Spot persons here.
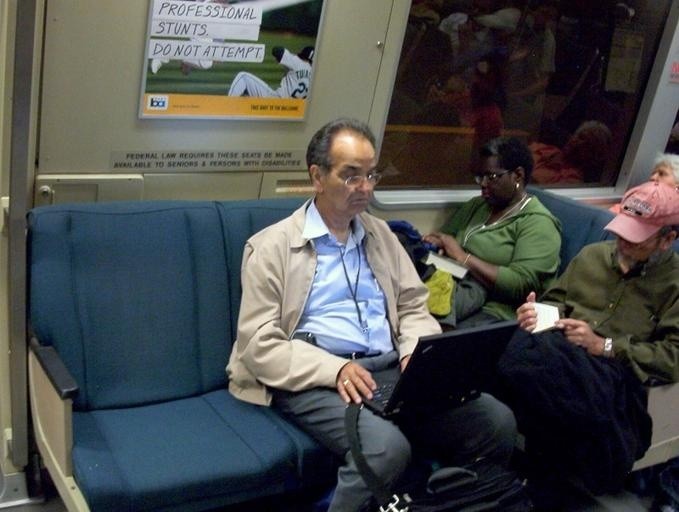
[490,180,679,497]
[227,44,313,100]
[648,152,679,189]
[217,116,525,508]
[412,134,566,334]
[378,0,632,188]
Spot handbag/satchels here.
[367,464,532,512]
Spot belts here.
[335,351,382,360]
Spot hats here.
[476,7,522,31]
[604,181,679,244]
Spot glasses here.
[473,169,513,184]
[327,164,382,189]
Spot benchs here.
[524,187,679,504]
[26,196,335,512]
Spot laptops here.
[362,320,521,420]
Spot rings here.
[342,378,350,386]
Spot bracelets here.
[603,334,613,358]
[462,252,472,266]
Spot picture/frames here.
[136,0,330,123]
